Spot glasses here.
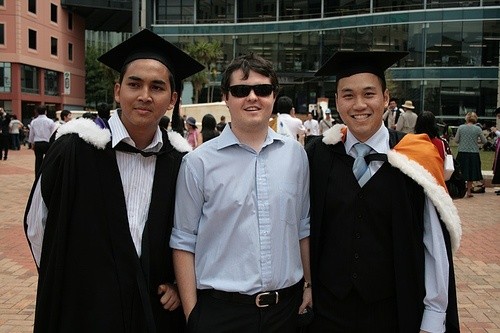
[227,83,277,98]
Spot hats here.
[313,50,411,81]
[97,27,206,84]
[185,117,196,126]
[401,100,415,109]
[325,108,331,113]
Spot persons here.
[0,108,23,160]
[305,50,463,333]
[33,29,206,333]
[269,96,340,148]
[170,50,313,333]
[161,114,227,149]
[491,107,500,195]
[382,98,445,164]
[455,112,487,198]
[28,103,110,178]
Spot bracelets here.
[304,281,312,288]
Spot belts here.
[196,277,306,309]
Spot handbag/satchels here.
[443,153,455,183]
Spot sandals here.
[470,183,486,194]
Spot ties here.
[350,143,374,187]
